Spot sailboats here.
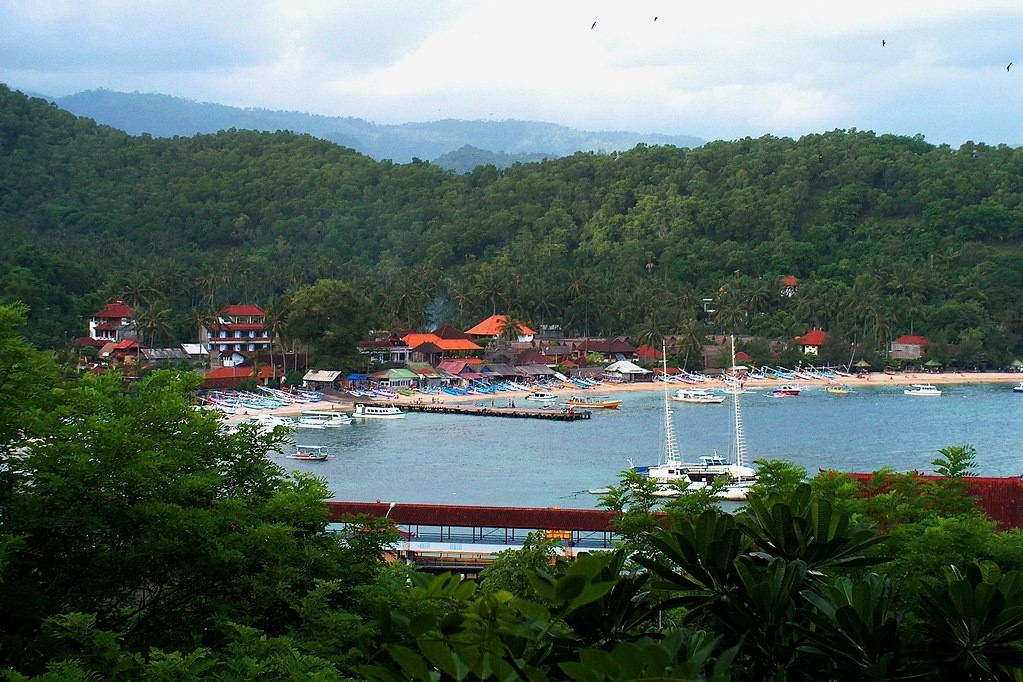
[628,333,772,500]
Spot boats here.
[200,385,354,439]
[762,384,800,399]
[524,391,558,402]
[652,348,857,384]
[1013,380,1023,392]
[587,488,614,494]
[884,369,897,375]
[671,386,727,403]
[351,404,408,419]
[286,444,329,460]
[904,383,941,397]
[825,384,851,394]
[718,385,747,395]
[564,395,622,409]
[559,401,605,412]
[345,374,601,398]
